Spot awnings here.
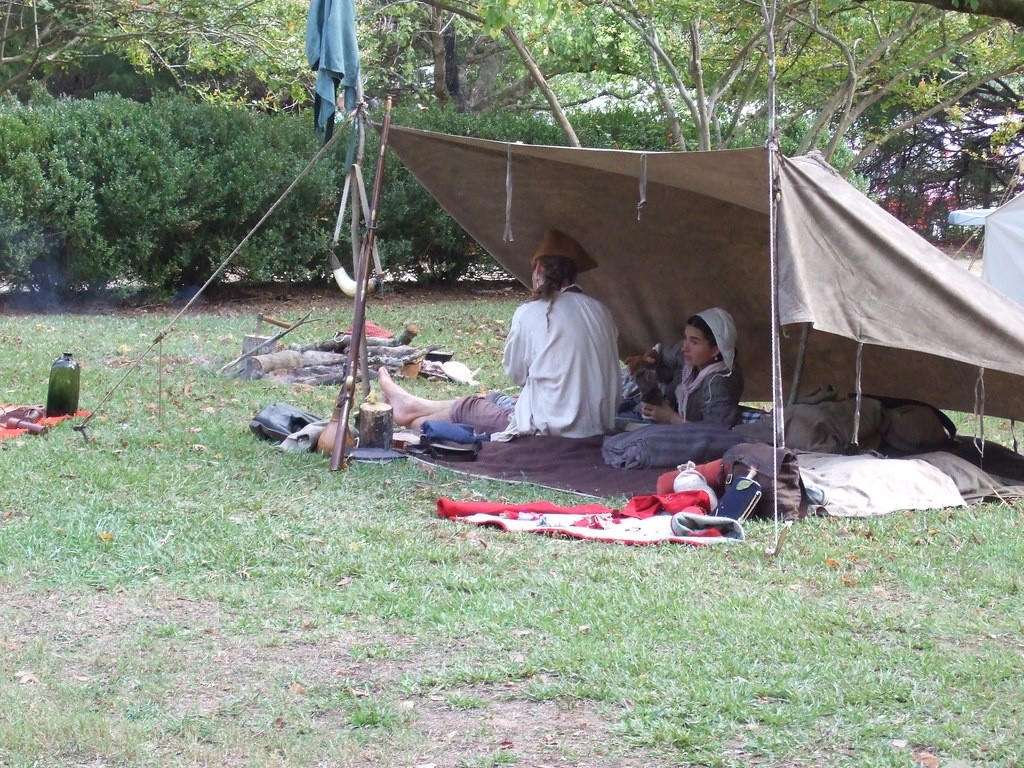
[72,102,1024,557]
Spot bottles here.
[45,352,80,418]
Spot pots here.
[426,351,455,363]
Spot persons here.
[617,307,744,430]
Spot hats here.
[531,229,598,272]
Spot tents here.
[377,230,621,442]
[948,188,1024,308]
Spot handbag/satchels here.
[717,442,808,522]
[249,403,321,443]
[277,418,359,456]
[392,420,482,462]
